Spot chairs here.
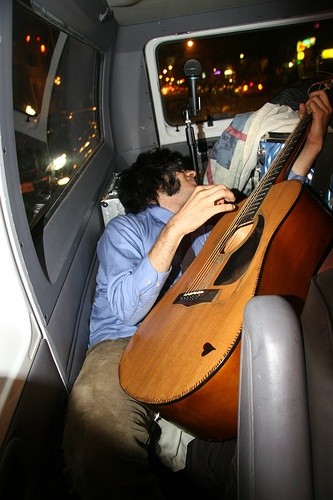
[236,267,333,500]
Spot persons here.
[56,89,333,500]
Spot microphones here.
[185,59,202,116]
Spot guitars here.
[118,81,332,442]
